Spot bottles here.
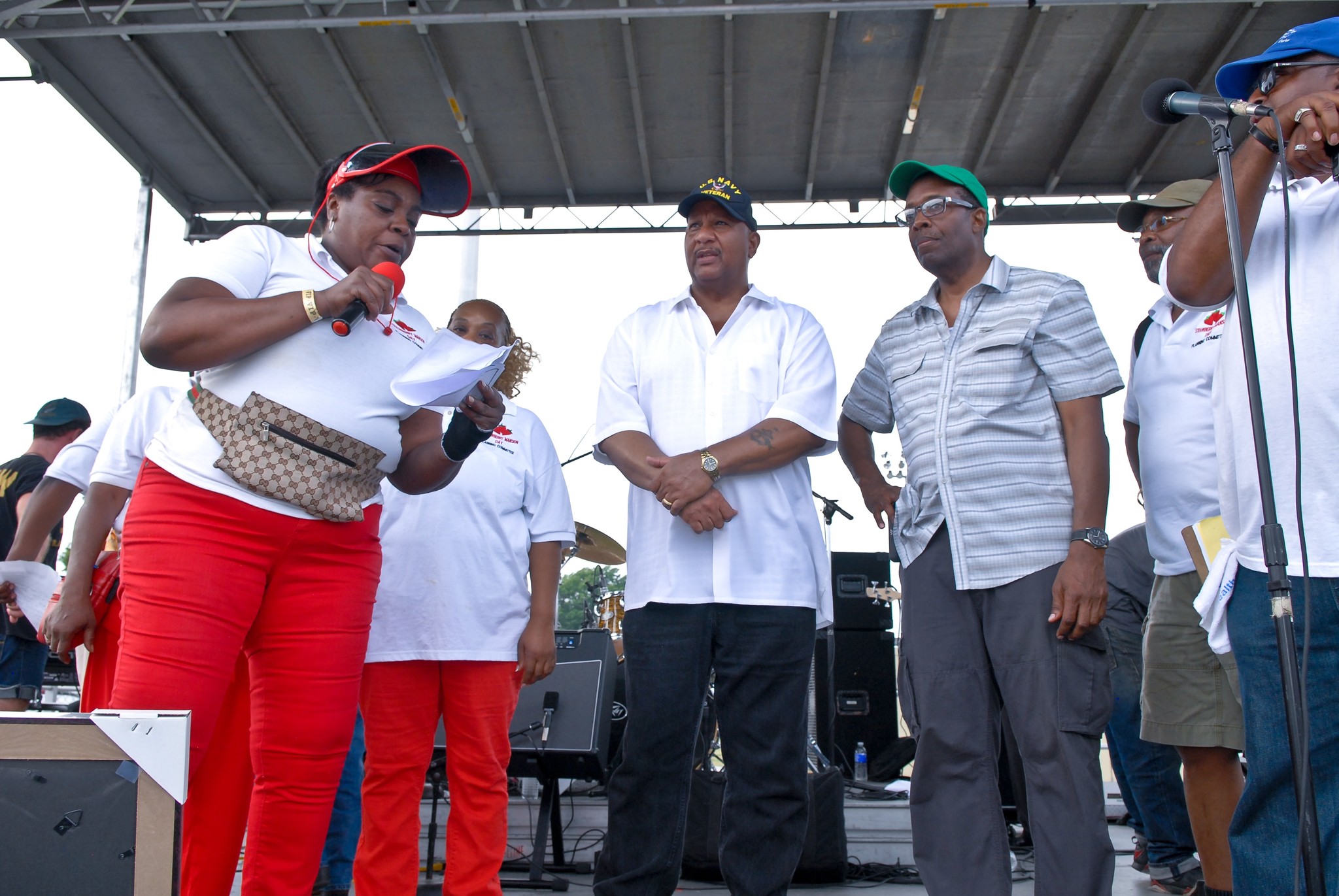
[855,742,868,782]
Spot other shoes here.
[1008,829,1035,848]
[1149,862,1206,893]
[1130,841,1151,874]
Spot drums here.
[607,652,718,771]
[594,589,625,659]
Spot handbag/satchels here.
[187,381,389,523]
[36,547,121,651]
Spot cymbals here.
[562,520,628,566]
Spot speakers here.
[813,630,901,781]
[831,552,894,632]
[688,770,847,883]
[434,629,615,779]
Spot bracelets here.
[1248,123,1287,155]
[302,289,323,323]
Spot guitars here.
[866,580,902,608]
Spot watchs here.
[1070,527,1109,549]
[700,447,720,481]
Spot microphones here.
[331,262,405,337]
[542,691,559,748]
[1140,79,1274,126]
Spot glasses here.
[1244,61,1339,101]
[1132,216,1187,243]
[895,196,973,227]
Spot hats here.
[888,160,989,234]
[23,398,91,426]
[327,141,472,217]
[1215,17,1339,101]
[1116,179,1213,232]
[677,175,757,231]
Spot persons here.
[1101,519,1206,896]
[1159,17,1339,896]
[109,142,505,896]
[1118,178,1248,896]
[837,160,1126,896]
[1,383,180,713]
[352,299,577,896]
[312,704,366,896]
[589,177,839,895]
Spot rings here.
[1294,144,1307,150]
[1294,107,1314,123]
[663,498,672,505]
